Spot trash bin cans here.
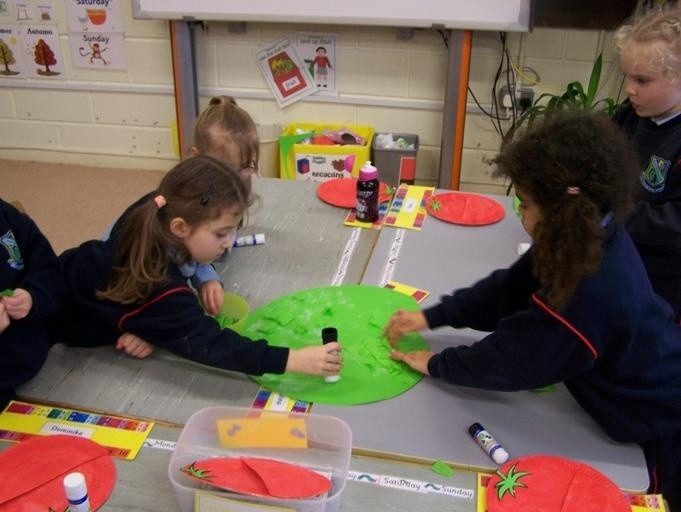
[372,131,419,185]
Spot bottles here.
[355,160,380,222]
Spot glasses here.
[239,160,258,179]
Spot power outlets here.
[499,88,534,110]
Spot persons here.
[0,195,61,417]
[48,155,344,379]
[189,95,259,195]
[599,8,681,316]
[380,105,678,512]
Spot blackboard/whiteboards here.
[131,0,536,33]
